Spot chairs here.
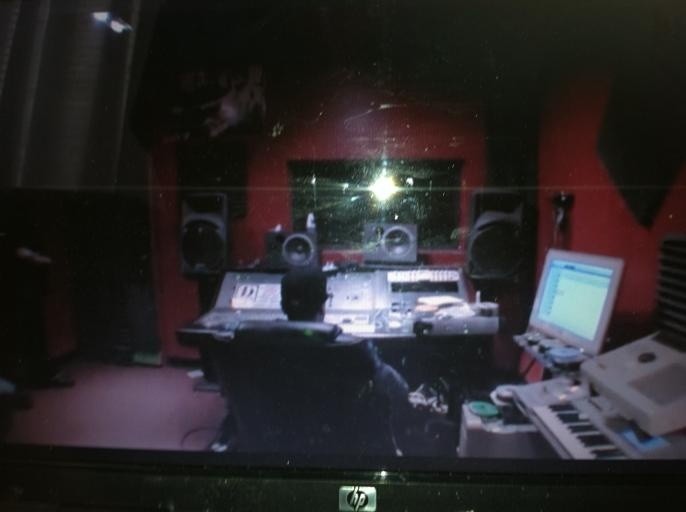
[230,338,368,455]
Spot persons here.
[272,268,408,431]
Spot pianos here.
[530,403,640,459]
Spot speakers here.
[267,228,322,272]
[363,223,419,265]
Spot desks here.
[514,330,577,460]
[178,326,495,400]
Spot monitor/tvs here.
[529,248,626,357]
[0,1,686,511]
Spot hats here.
[281,267,327,313]
